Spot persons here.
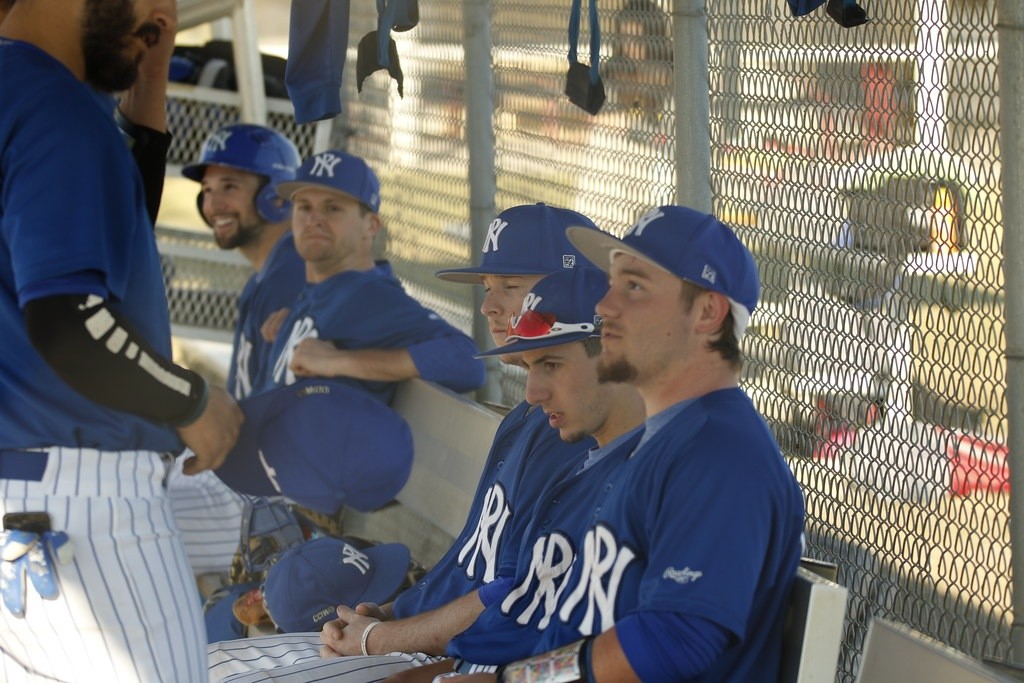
[433,208,803,682]
[389,272,648,683]
[0,0,246,683]
[167,149,484,576]
[181,123,302,402]
[207,201,601,679]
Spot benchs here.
[197,376,850,683]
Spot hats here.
[263,536,410,633]
[435,201,600,284]
[471,265,611,360]
[273,149,381,213]
[564,205,761,342]
[213,377,414,516]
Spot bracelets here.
[361,621,384,657]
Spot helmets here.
[181,124,302,229]
